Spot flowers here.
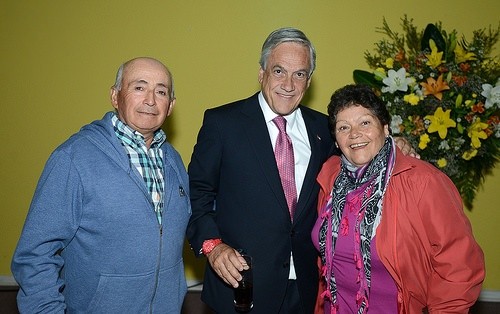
[351,14,500,208]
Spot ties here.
[272,115,298,223]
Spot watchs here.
[202,239,222,254]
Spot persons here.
[311,82,487,314]
[12,57,216,314]
[188,27,420,314]
